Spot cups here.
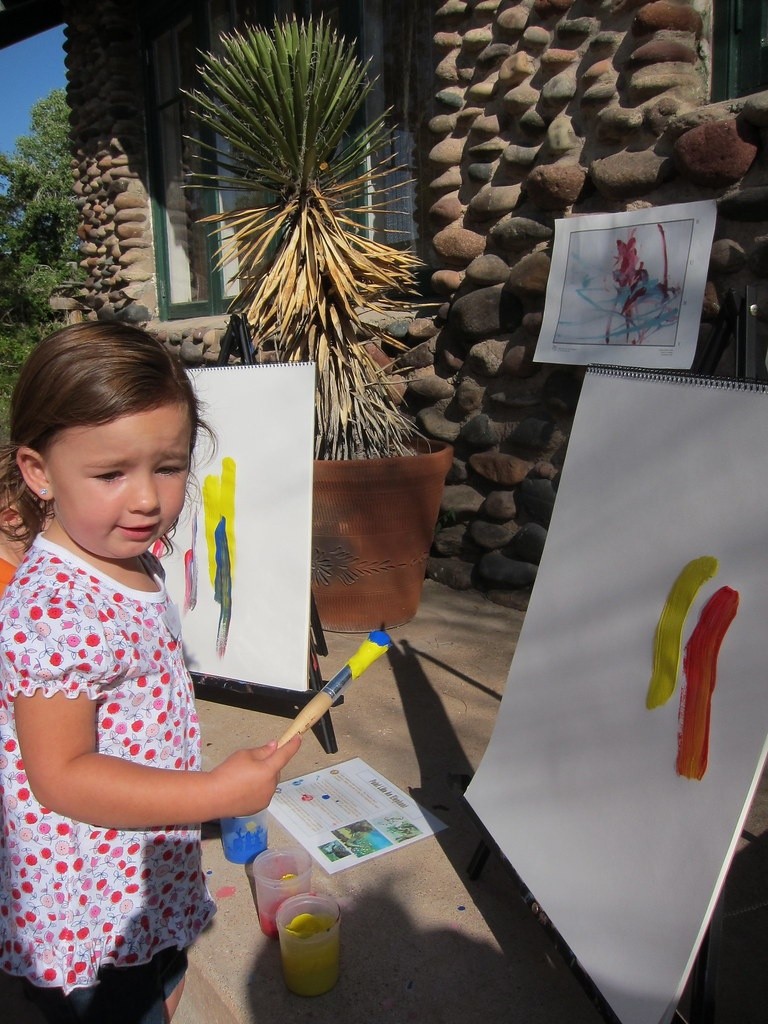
[275,892,343,997]
[219,807,270,864]
[252,846,313,940]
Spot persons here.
[0,319,304,1024]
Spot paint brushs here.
[276,632,392,748]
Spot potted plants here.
[175,10,452,631]
